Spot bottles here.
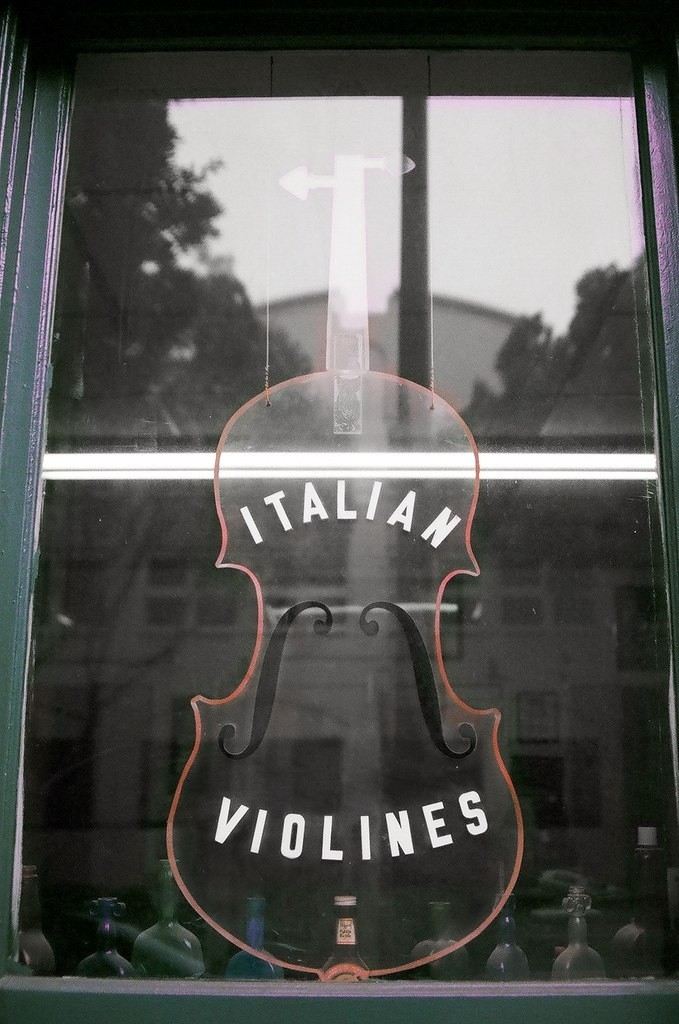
[132,856,206,981]
[316,895,376,983]
[406,901,475,983]
[222,896,285,980]
[605,828,679,981]
[483,892,533,984]
[75,896,137,980]
[550,884,609,980]
[17,864,57,977]
